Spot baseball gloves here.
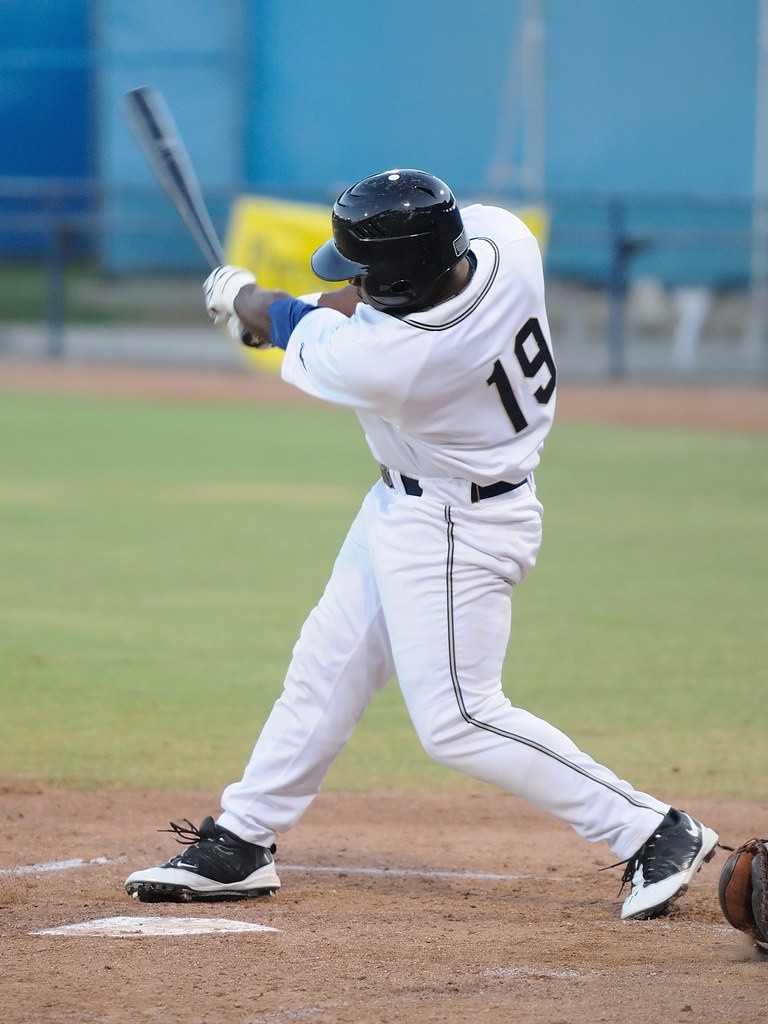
[717,836,768,950]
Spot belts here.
[379,463,527,502]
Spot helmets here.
[311,169,470,306]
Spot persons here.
[126,171,720,920]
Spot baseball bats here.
[125,84,268,349]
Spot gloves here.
[203,264,255,324]
[222,313,276,349]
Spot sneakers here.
[125,816,281,902]
[599,808,720,920]
